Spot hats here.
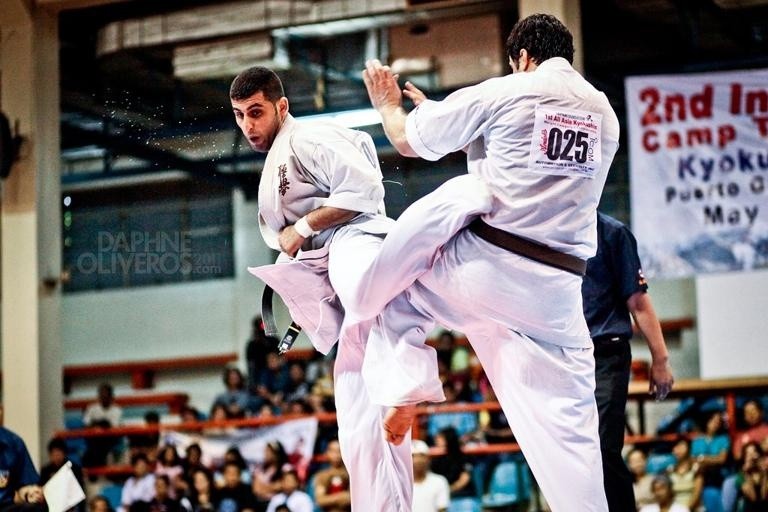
[408,439,432,458]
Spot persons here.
[362,13,621,512]
[36,315,352,512]
[625,399,767,512]
[0,397,42,511]
[230,67,494,512]
[582,208,673,511]
[406,352,530,512]
